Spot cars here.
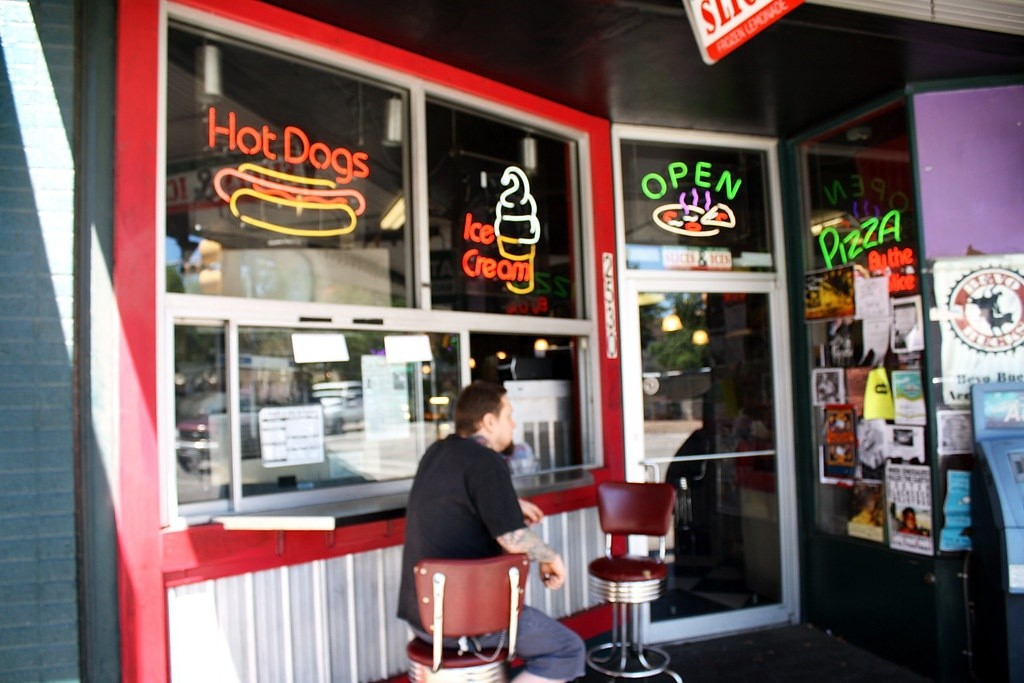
[311,381,365,437]
[179,395,288,465]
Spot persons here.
[665,394,719,561]
[395,382,586,683]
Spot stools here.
[405,554,530,683]
[585,482,683,683]
